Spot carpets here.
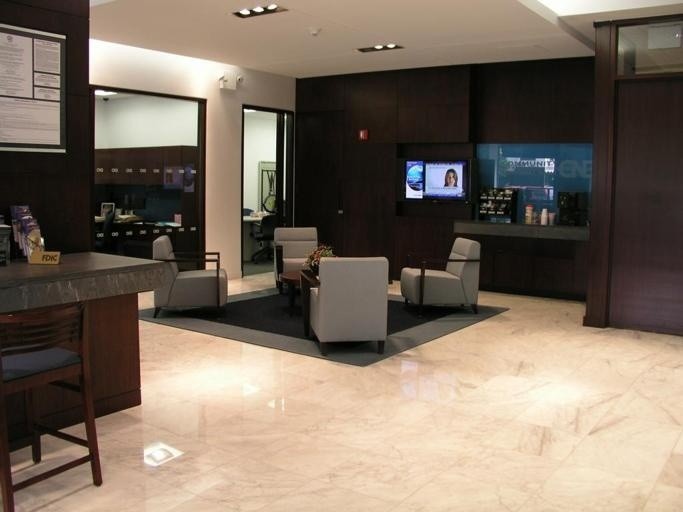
[138,282,511,368]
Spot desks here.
[452,218,589,299]
[243,216,263,262]
[1,247,174,436]
[278,269,309,317]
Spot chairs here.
[248,215,282,265]
[0,303,104,512]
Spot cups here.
[547,212,555,224]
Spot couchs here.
[297,255,393,355]
[149,233,228,321]
[271,226,318,278]
[398,237,486,317]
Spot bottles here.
[525,205,540,224]
[541,207,547,225]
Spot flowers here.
[303,245,335,266]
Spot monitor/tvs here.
[397,159,471,203]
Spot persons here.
[444,168,458,187]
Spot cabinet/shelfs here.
[94,222,197,259]
[94,144,195,189]
